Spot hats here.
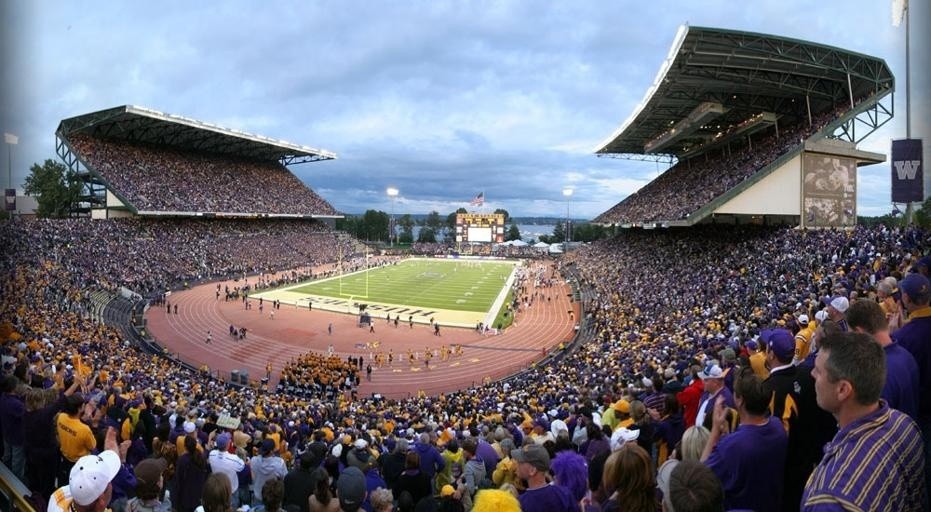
[69,449,122,506]
[133,457,169,485]
[610,426,641,454]
[337,465,367,509]
[759,328,797,357]
[795,301,829,325]
[642,360,690,388]
[509,444,550,471]
[216,432,232,450]
[819,293,851,314]
[718,340,758,364]
[897,272,930,296]
[695,364,724,381]
[5,337,630,441]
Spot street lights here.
[387,185,400,250]
[3,130,19,189]
[890,2,918,229]
[563,184,575,243]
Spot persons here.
[1,91,931,512]
